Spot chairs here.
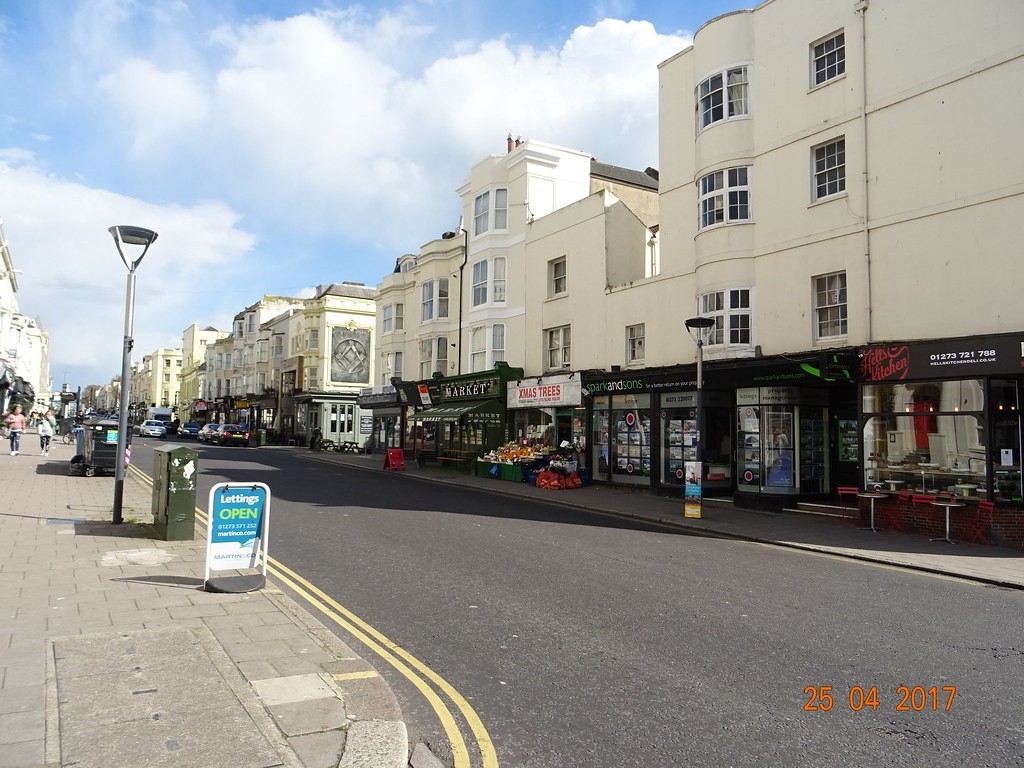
[879,489,939,539]
[957,502,996,546]
[838,486,864,526]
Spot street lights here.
[107,224,159,523]
[684,316,717,519]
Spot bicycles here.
[62,427,75,446]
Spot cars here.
[211,423,251,448]
[176,422,201,439]
[196,422,220,442]
[138,419,168,437]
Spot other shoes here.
[16,450,19,455]
[9,451,14,456]
[45,453,48,457]
[41,449,44,456]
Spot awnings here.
[408,399,505,470]
[183,399,207,410]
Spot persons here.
[74,410,84,439]
[29,411,37,428]
[38,411,56,457]
[309,426,323,449]
[39,411,44,420]
[4,404,25,456]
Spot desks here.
[857,493,890,533]
[321,439,336,451]
[927,501,967,545]
[439,450,478,473]
[343,441,361,454]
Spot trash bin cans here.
[81,417,133,477]
[256,429,266,445]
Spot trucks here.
[147,407,177,434]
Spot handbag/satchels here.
[38,423,43,434]
[4,426,11,437]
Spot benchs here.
[437,455,475,473]
[319,444,363,451]
